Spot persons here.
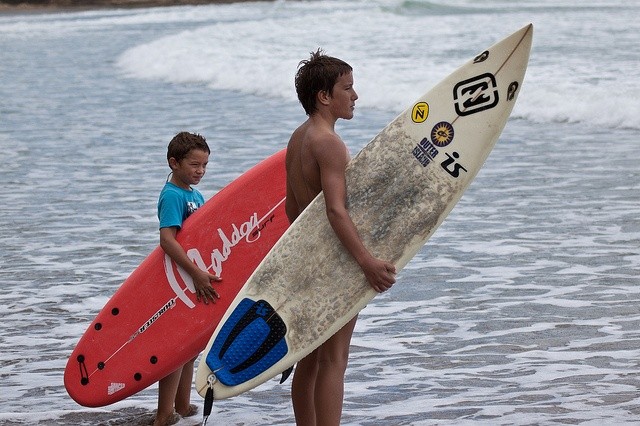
[284,47,397,426]
[153,131,222,425]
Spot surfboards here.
[63,148,290,408]
[195,22,534,399]
